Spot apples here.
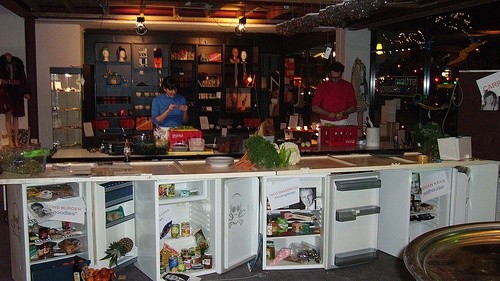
[87,267,116,281]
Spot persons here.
[312,62,357,125]
[0,52,30,149]
[299,188,316,211]
[230,48,247,63]
[30,202,55,223]
[116,47,126,61]
[482,90,498,111]
[151,76,190,143]
[411,173,422,195]
[102,47,110,61]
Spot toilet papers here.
[394,99,400,109]
[381,106,395,122]
[160,127,172,140]
[366,128,379,147]
[385,100,396,106]
[380,124,386,136]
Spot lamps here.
[234,17,248,37]
[134,15,149,37]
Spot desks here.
[50,146,245,162]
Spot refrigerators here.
[259,172,382,270]
[8,182,138,281]
[378,162,500,258]
[134,177,258,281]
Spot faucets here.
[122,128,131,162]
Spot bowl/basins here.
[119,118,135,130]
[206,157,233,168]
[92,120,110,133]
[403,221,500,281]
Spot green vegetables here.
[178,126,193,130]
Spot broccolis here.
[273,142,301,165]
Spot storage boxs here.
[436,135,473,161]
[120,119,134,129]
[243,118,259,128]
[318,125,359,147]
[153,57,163,69]
[135,116,153,130]
[167,129,203,150]
[187,52,195,60]
[188,137,205,151]
[179,49,188,60]
[92,120,110,131]
[208,52,222,62]
[138,57,148,67]
[379,98,406,144]
[138,47,147,57]
[217,118,234,126]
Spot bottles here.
[171,45,193,87]
[119,48,125,62]
[135,114,153,130]
[102,47,109,61]
[106,72,122,86]
[196,71,223,100]
[134,91,161,111]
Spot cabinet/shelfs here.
[49,66,85,149]
[0,150,500,281]
[93,41,308,134]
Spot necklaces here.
[8,60,12,64]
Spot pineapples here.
[100,238,133,268]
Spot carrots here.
[232,151,256,171]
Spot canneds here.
[35,243,44,260]
[272,221,278,235]
[39,226,48,240]
[171,224,180,238]
[204,253,212,269]
[159,183,175,197]
[182,256,191,270]
[181,221,190,237]
